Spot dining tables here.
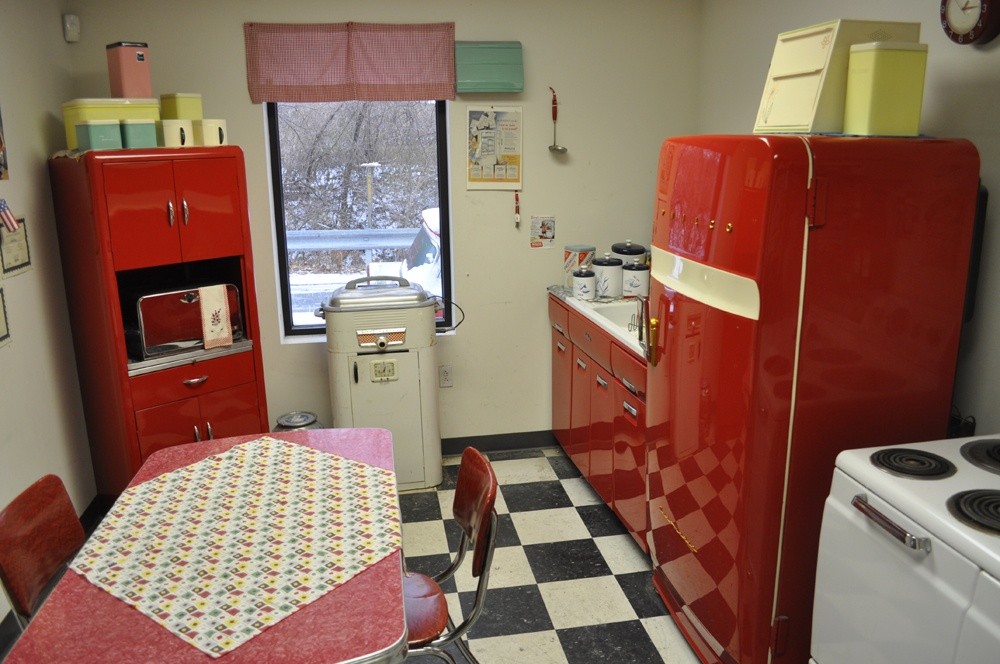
[2,429,410,662]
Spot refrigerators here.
[640,101,978,663]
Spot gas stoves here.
[835,433,999,582]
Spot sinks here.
[566,295,649,360]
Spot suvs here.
[398,207,445,322]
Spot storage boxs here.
[752,18,929,137]
[60,40,227,152]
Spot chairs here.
[404,445,499,664]
[1,473,86,636]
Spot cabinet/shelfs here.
[546,286,647,555]
[45,148,271,511]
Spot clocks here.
[937,1,1000,44]
